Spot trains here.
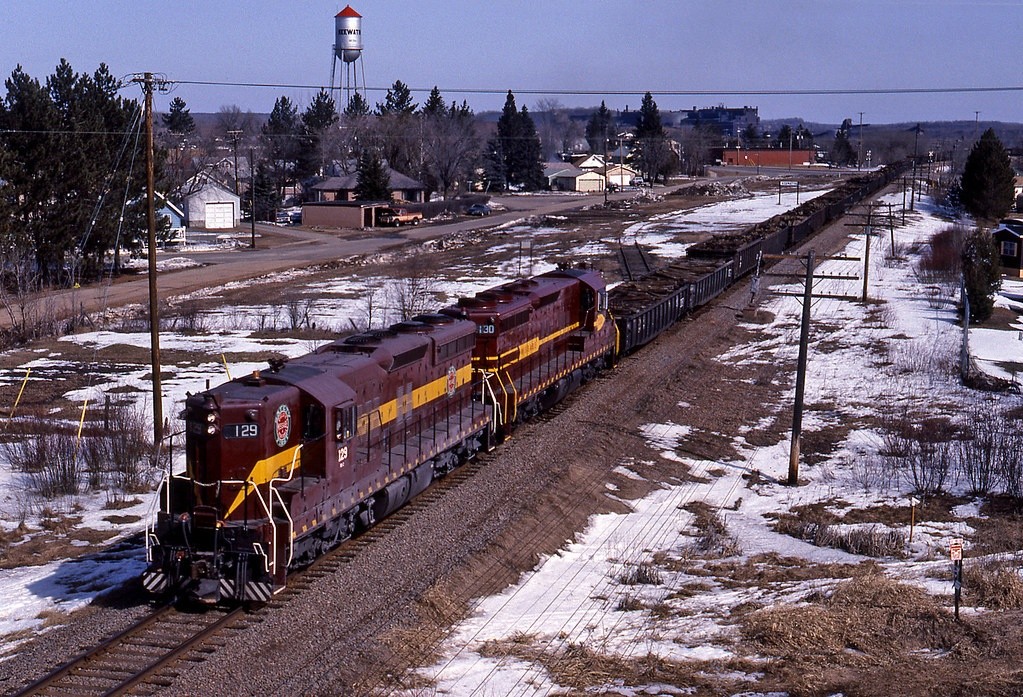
[132,155,947,611]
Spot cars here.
[290,212,302,223]
[275,212,290,223]
[467,204,492,216]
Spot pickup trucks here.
[381,208,423,227]
[629,177,649,186]
